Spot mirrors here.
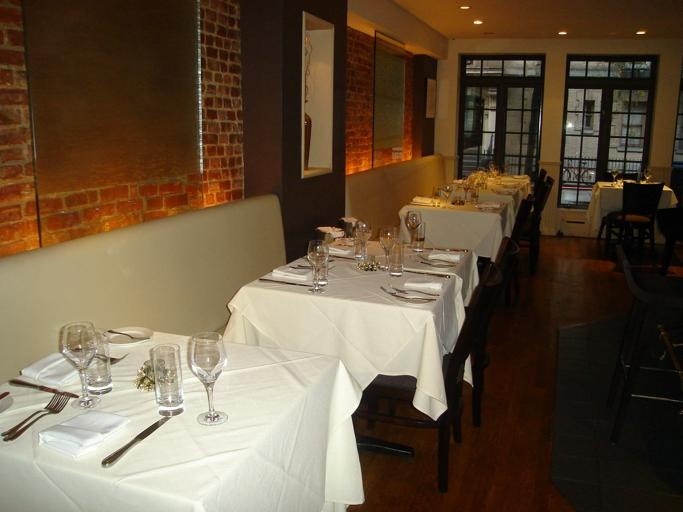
[18,1,205,195]
[370,27,410,154]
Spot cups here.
[85,336,114,394]
[150,340,184,417]
[431,163,502,207]
[413,224,425,253]
[389,237,405,276]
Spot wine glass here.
[187,330,225,425]
[609,167,655,188]
[58,320,100,409]
[295,209,426,294]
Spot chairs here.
[588,168,683,465]
[491,153,557,313]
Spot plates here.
[104,327,155,347]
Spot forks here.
[107,330,149,340]
[2,390,71,445]
[92,349,129,366]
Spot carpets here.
[551,311,682,507]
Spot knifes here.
[95,412,174,467]
[8,379,79,399]
[258,277,314,290]
